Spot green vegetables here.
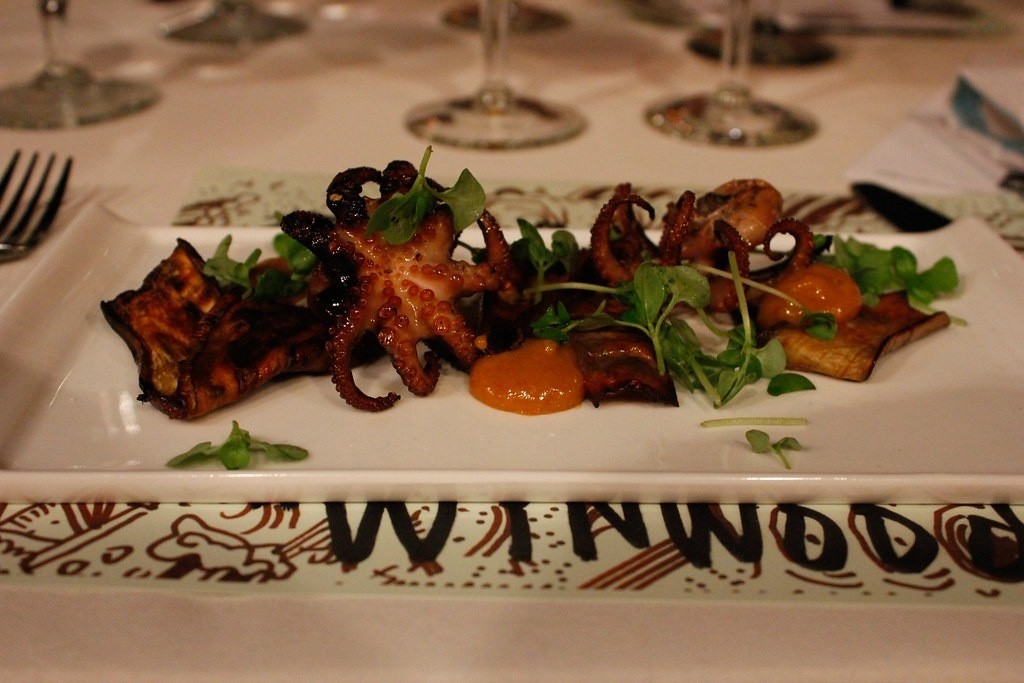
[165,145,973,474]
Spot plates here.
[0,203,1024,505]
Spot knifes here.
[851,181,953,233]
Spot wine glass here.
[646,1,820,149]
[405,0,588,150]
[442,0,569,33]
[689,0,837,68]
[167,0,307,46]
[1,0,157,129]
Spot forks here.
[0,147,74,265]
